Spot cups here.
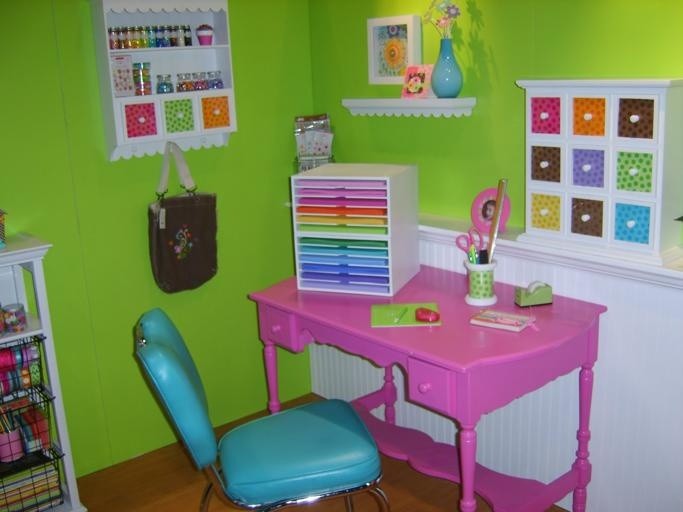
[0,432,22,463]
[196,30,212,45]
[462,258,497,301]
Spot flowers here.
[420,2,464,41]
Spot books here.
[0,455,64,512]
[294,179,389,292]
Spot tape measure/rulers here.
[487,180,506,263]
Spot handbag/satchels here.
[148,193,218,293]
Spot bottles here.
[176,69,223,93]
[132,61,152,96]
[107,23,192,49]
[1,304,28,333]
[156,73,173,93]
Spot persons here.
[483,201,495,222]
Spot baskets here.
[0,334,65,512]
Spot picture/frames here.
[363,10,421,88]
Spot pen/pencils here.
[395,308,407,322]
[469,245,476,264]
[0,406,14,434]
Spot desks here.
[241,264,609,511]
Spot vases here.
[427,37,465,98]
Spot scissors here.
[456,227,483,255]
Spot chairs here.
[128,307,395,512]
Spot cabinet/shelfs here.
[2,230,91,512]
[287,162,423,298]
[510,75,680,268]
[91,2,237,164]
